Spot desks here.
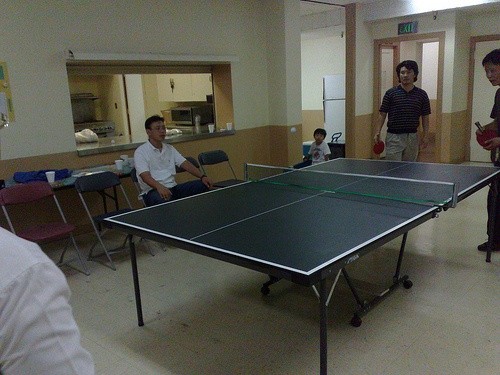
[5,165,120,229]
[102,156,500,375]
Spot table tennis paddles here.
[373,134,385,154]
[475,121,496,147]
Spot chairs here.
[0,180,90,277]
[74,171,154,271]
[176,157,201,173]
[198,150,247,187]
[132,167,166,252]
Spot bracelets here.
[201,175,207,181]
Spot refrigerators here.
[322,74,344,141]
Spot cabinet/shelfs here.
[156,73,212,102]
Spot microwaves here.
[169,105,212,126]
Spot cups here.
[120,155,128,165]
[208,125,214,133]
[45,172,55,183]
[226,123,232,130]
[114,160,123,170]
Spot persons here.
[302,128,332,166]
[133,115,215,207]
[0,226,95,375]
[372,59,432,162]
[475,48,500,252]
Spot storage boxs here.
[302,140,314,156]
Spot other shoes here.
[478,241,500,251]
